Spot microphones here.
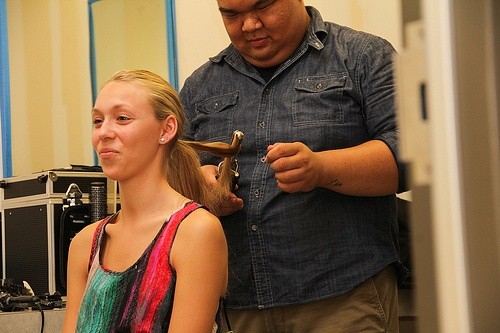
[23,279,42,312]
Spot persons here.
[176,0,409,333]
[62,69,230,333]
